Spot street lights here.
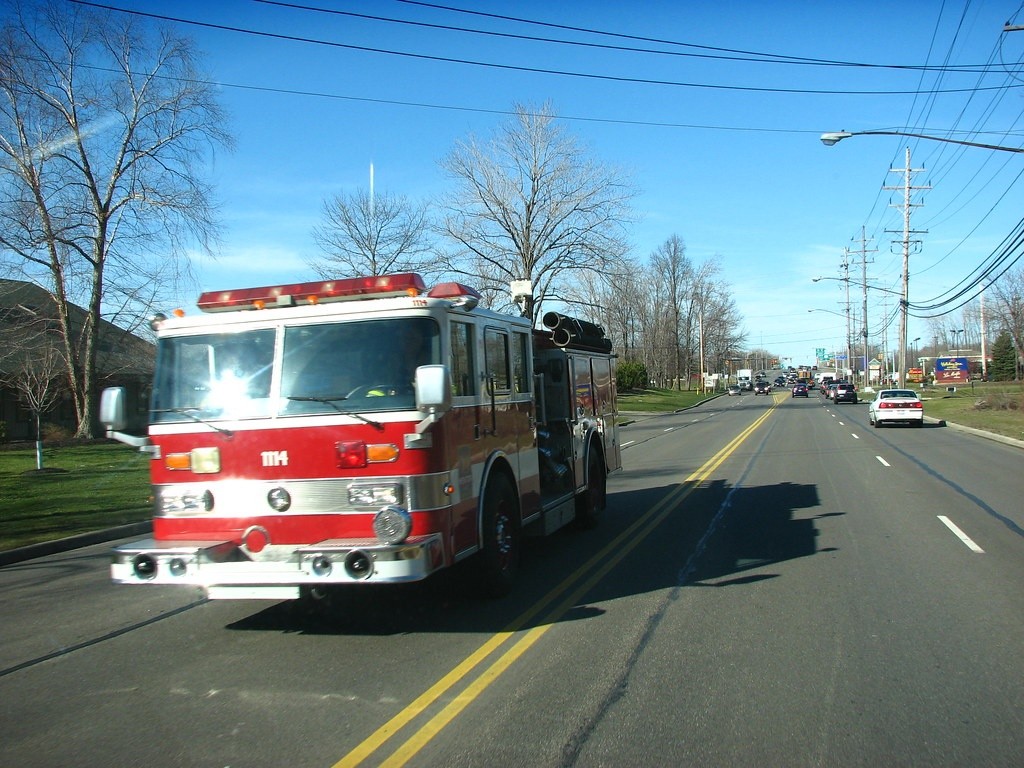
[950,329,964,356]
[812,277,905,390]
[808,307,870,392]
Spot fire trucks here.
[97,272,626,627]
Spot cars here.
[833,384,857,405]
[727,364,848,399]
[868,389,923,428]
